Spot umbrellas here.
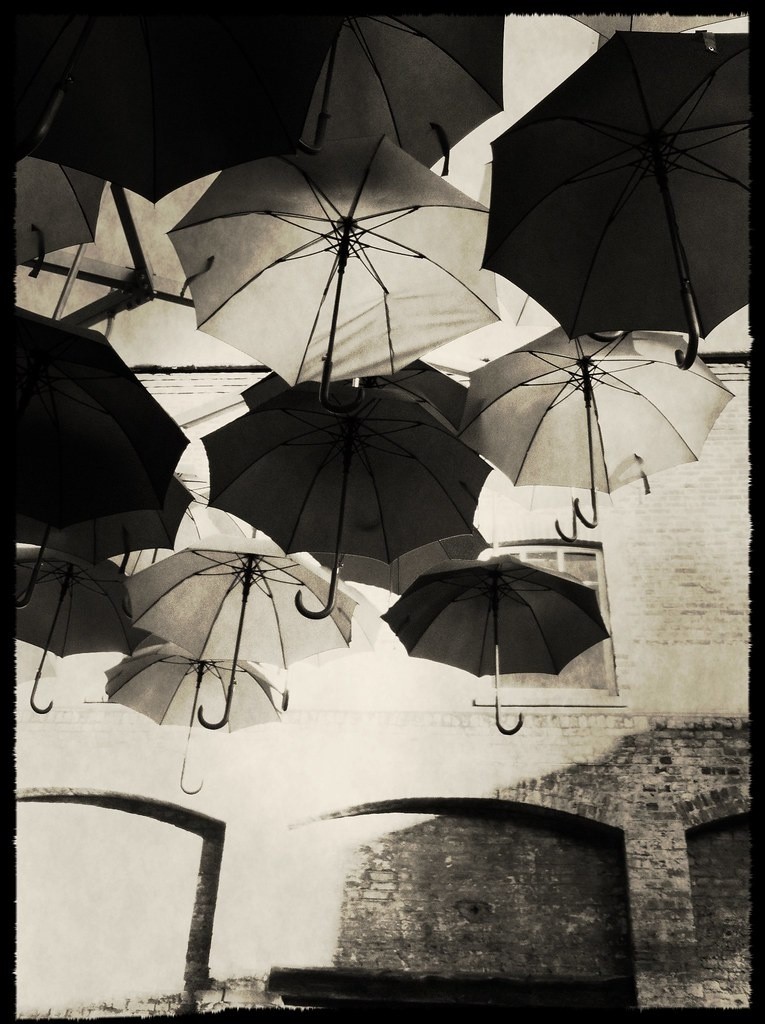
[13,13,506,342]
[202,359,494,620]
[12,302,361,795]
[457,326,734,542]
[381,557,609,734]
[483,15,750,368]
[167,132,500,414]
